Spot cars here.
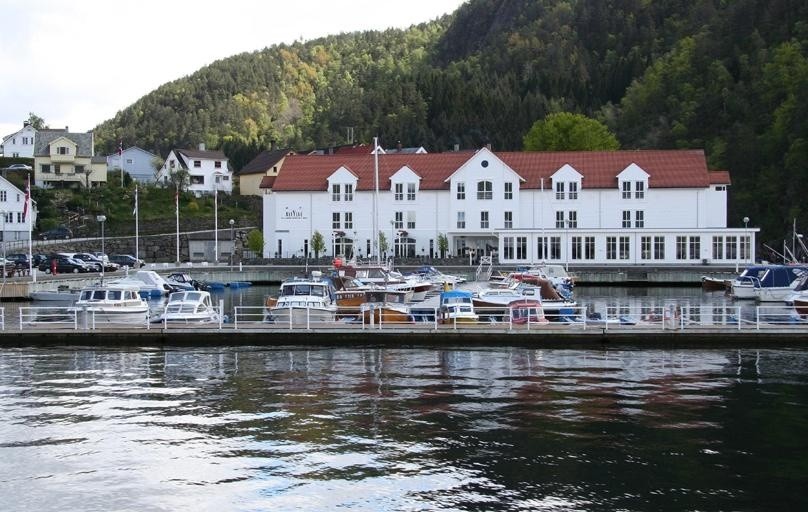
[0,251,146,277]
[38,228,73,241]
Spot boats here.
[28,270,252,323]
[700,217,807,320]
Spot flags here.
[24,174,31,222]
[131,184,137,216]
[114,136,122,158]
[174,185,178,216]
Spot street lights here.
[100,214,107,274]
[391,219,395,272]
[742,217,749,270]
[566,219,569,273]
[229,218,235,266]
